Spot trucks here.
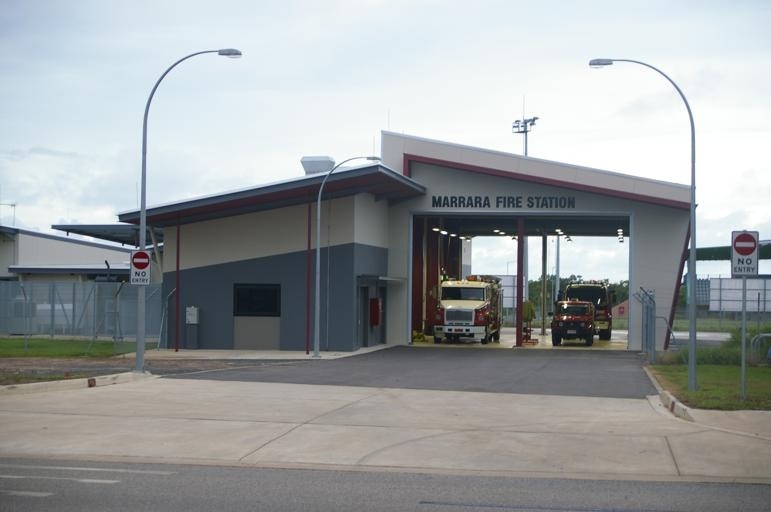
[563,280,616,339]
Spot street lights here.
[132,47,246,374]
[511,116,539,156]
[310,154,382,361]
[587,56,701,397]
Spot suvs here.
[548,301,596,348]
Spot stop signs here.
[732,233,757,258]
[130,251,151,270]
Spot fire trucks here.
[431,275,505,344]
